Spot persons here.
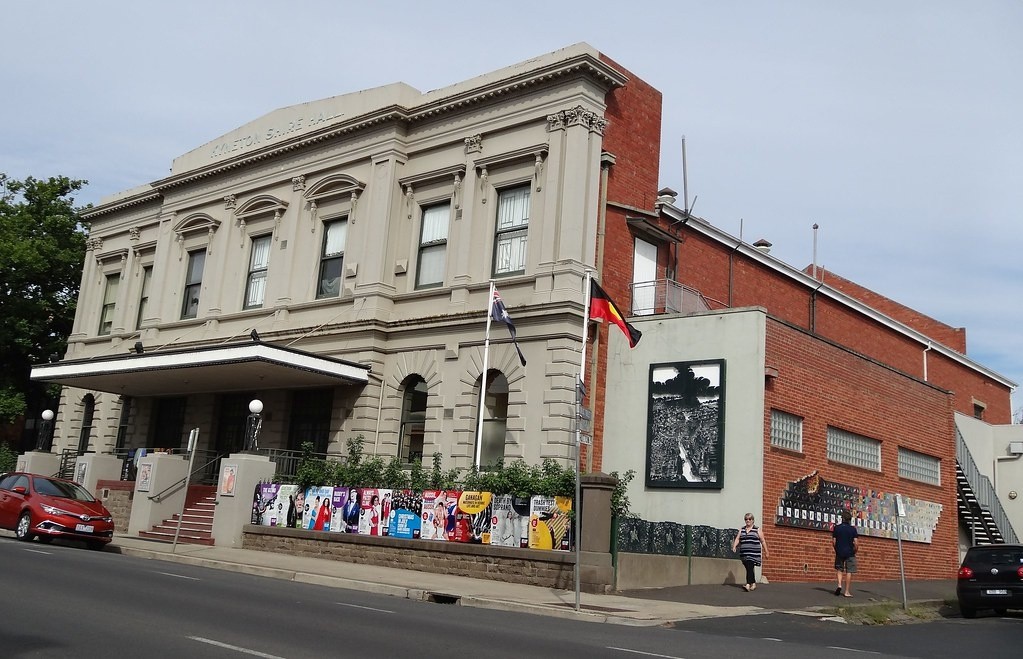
[732,512,769,592]
[832,512,858,596]
[256,489,514,546]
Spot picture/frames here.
[645,358,727,490]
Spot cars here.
[0,472,115,550]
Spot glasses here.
[745,518,752,521]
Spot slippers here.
[845,591,854,597]
[836,587,842,596]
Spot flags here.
[588,277,642,349]
[492,289,527,366]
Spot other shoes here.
[742,585,750,592]
[751,586,757,591]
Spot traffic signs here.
[580,379,587,397]
[579,419,593,446]
[579,405,592,420]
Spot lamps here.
[243,399,263,455]
[250,329,260,341]
[33,410,54,453]
[135,342,144,353]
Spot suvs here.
[956,543,1023,619]
